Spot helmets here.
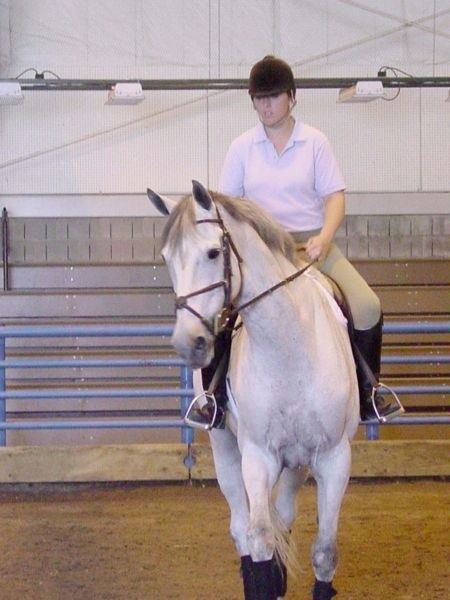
[248,55,294,97]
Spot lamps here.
[0,82,25,105]
[335,81,383,105]
[105,82,146,106]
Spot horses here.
[146,179,360,600]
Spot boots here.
[350,310,401,422]
[188,304,239,429]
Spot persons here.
[188,56,401,429]
